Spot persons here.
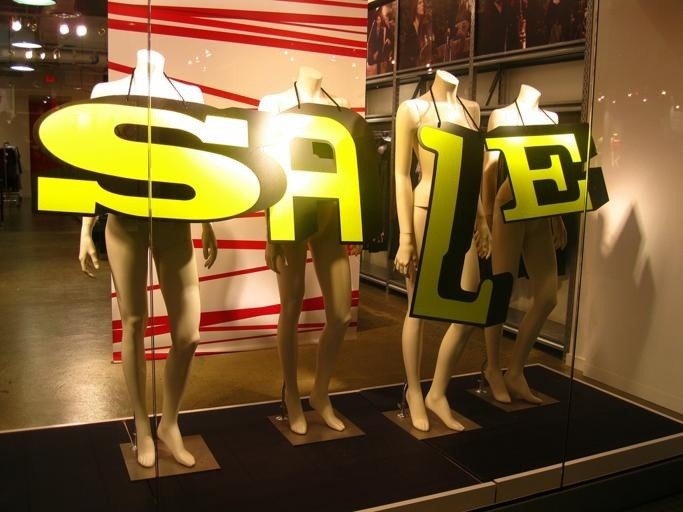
[472,82,570,406]
[252,65,356,437]
[388,65,494,434]
[367,1,576,76]
[69,50,200,471]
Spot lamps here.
[0,0,87,72]
